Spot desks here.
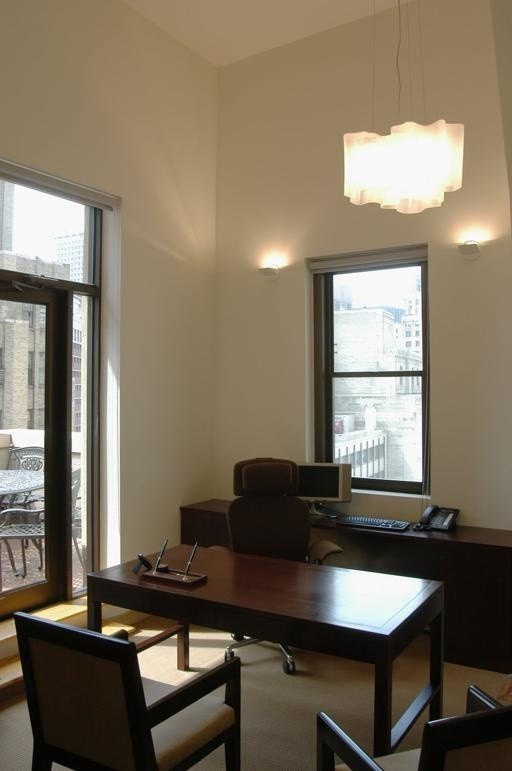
[87,544,442,758]
[179,498,512,674]
[0,469,45,551]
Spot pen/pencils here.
[184,538,199,578]
[153,537,169,575]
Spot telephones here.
[412,503,459,532]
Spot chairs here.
[316,684,512,771]
[0,469,83,577]
[2,445,44,548]
[220,495,343,674]
[15,611,242,771]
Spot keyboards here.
[335,516,411,532]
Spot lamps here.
[344,0,465,214]
[259,265,280,279]
[458,240,481,258]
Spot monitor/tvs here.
[295,463,352,518]
